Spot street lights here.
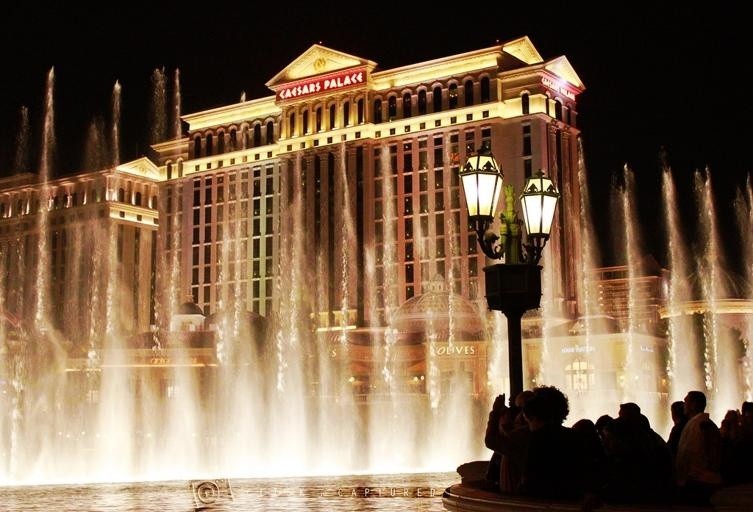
[459,146,562,412]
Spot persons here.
[482,383,752,512]
